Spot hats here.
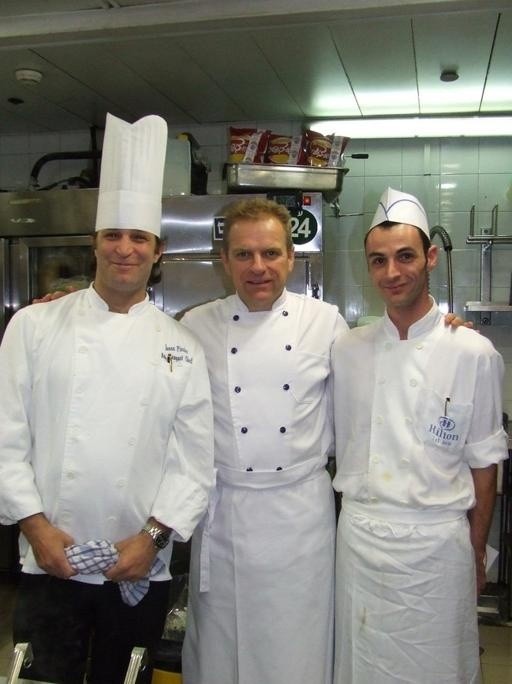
[368,186,431,242]
[96,111,170,241]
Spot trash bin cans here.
[152,574,189,684]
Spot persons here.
[31,196,482,684]
[328,220,510,684]
[0,227,217,684]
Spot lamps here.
[307,110,512,140]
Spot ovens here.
[1,189,97,325]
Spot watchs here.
[143,522,170,548]
